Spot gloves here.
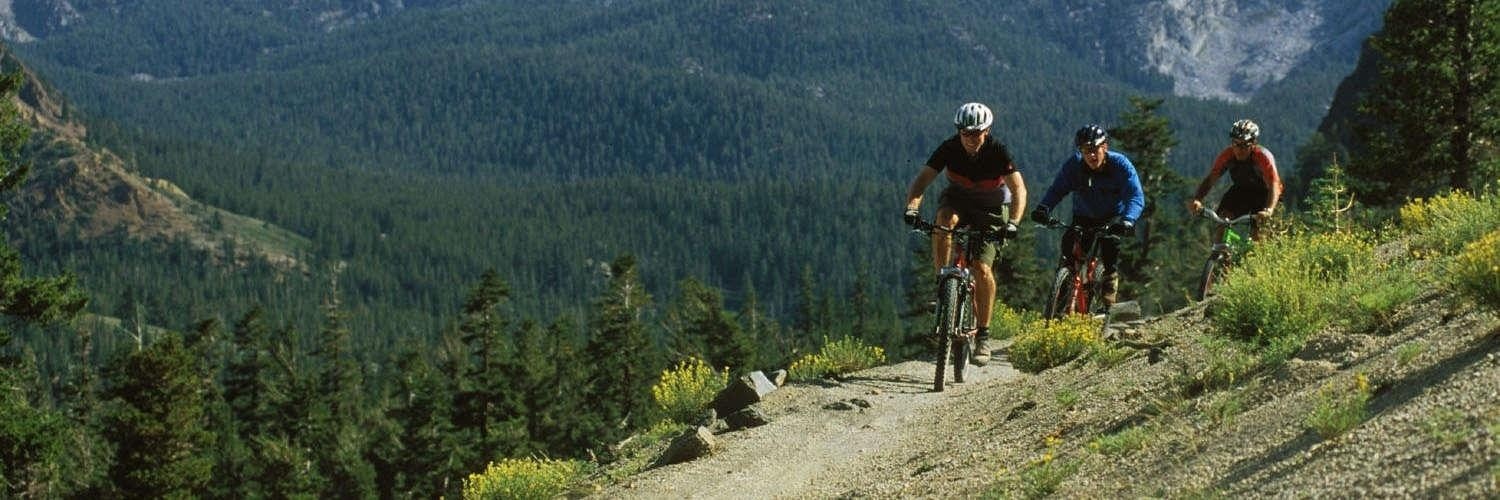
[1003,223,1018,238]
[1032,207,1047,223]
[1257,208,1272,226]
[1107,224,1136,237]
[904,208,918,224]
[1189,198,1201,217]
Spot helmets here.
[1074,124,1107,146]
[954,102,993,132]
[1230,119,1259,140]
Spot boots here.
[1102,273,1118,304]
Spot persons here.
[1031,121,1146,318]
[905,99,1029,368]
[1185,118,1284,267]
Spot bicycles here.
[915,214,1006,392]
[1197,205,1259,301]
[1042,214,1123,340]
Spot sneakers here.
[926,287,939,311]
[970,337,991,367]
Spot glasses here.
[1078,147,1098,153]
[1232,140,1252,147]
[960,133,982,136]
